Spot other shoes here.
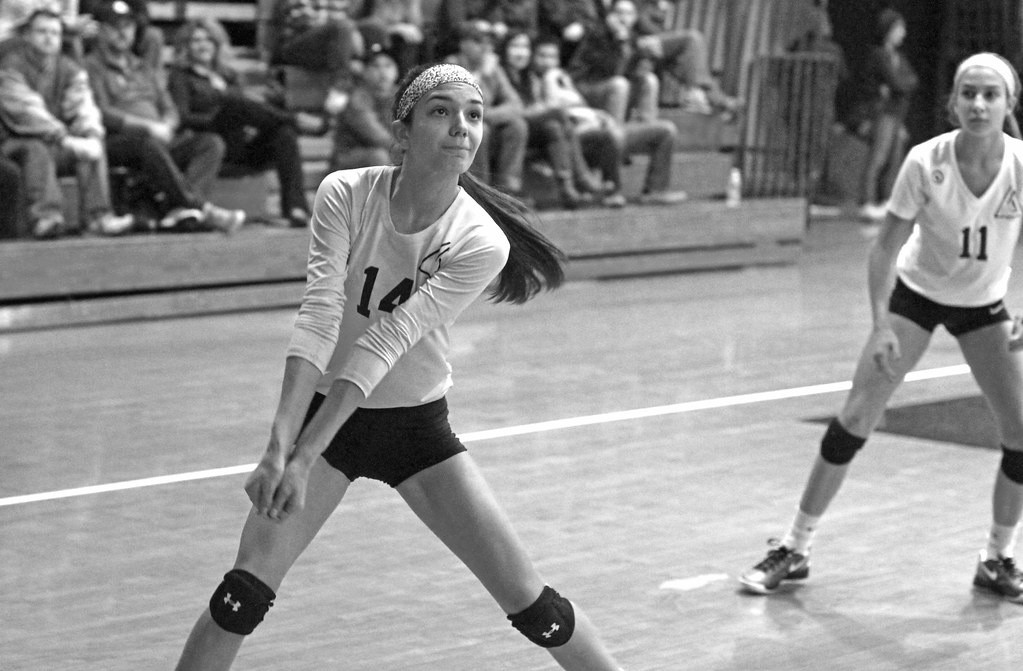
[577,173,614,196]
[600,192,626,208]
[158,205,205,232]
[295,112,330,137]
[29,213,68,239]
[640,188,687,204]
[853,199,889,221]
[559,187,593,209]
[287,205,309,228]
[81,210,137,237]
[200,199,247,233]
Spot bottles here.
[726,166,742,207]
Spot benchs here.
[621,108,732,195]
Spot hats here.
[92,1,138,22]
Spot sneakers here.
[972,548,1023,603]
[736,538,811,595]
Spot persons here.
[739,53,1022,605]
[170,63,631,671]
[0,0,917,242]
